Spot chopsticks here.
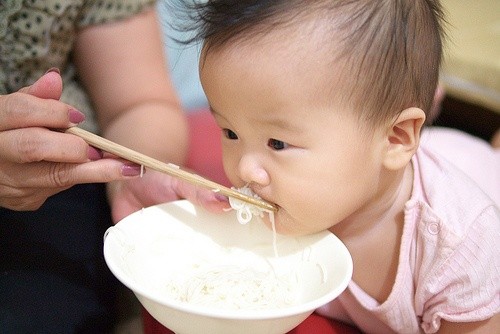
[51,125,279,213]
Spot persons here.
[0,0,232,334]
[178,0,500,334]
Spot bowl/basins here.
[102,200,353,334]
